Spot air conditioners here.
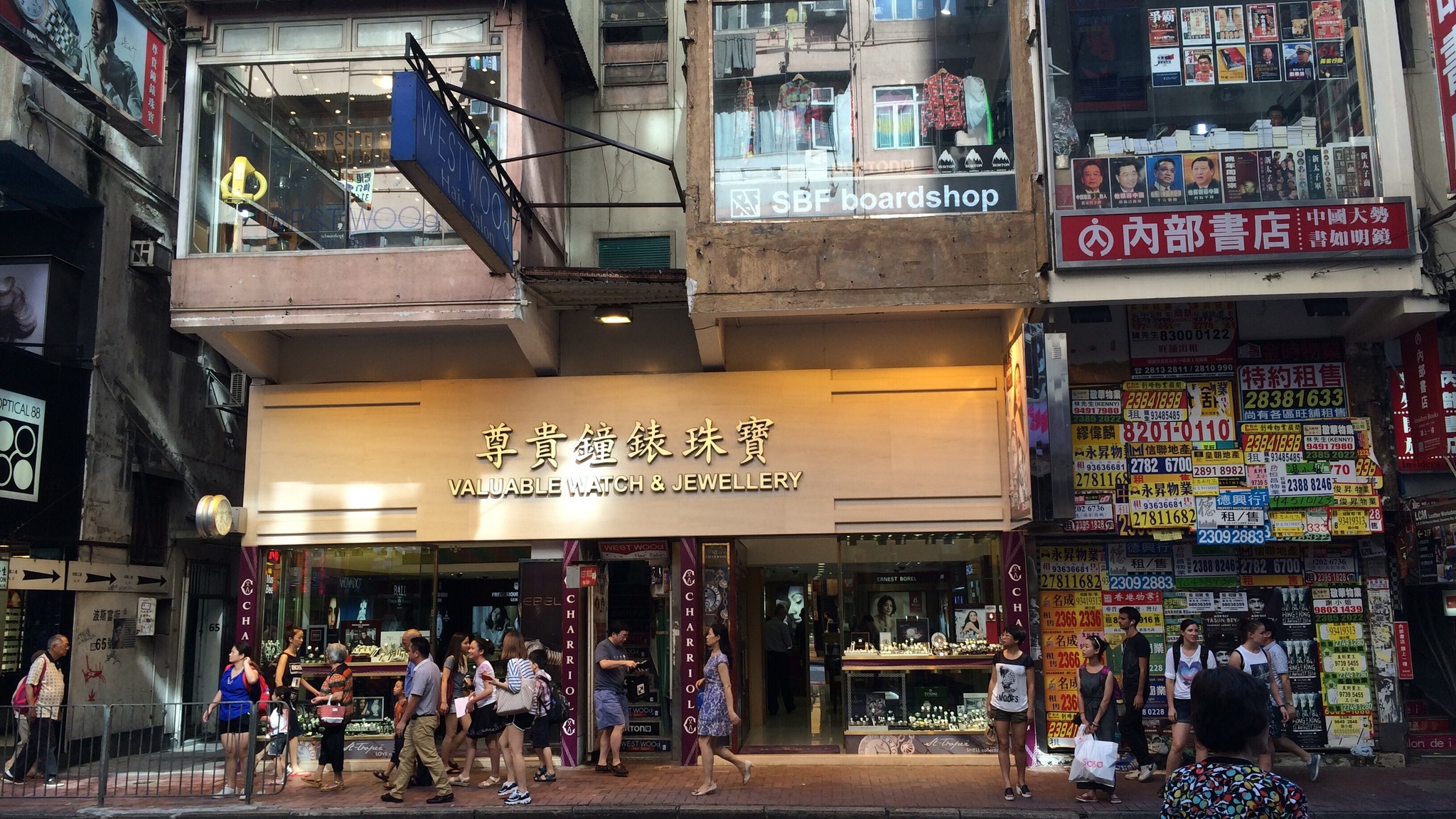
[130,240,173,277]
[230,372,249,409]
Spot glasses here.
[1088,635,1101,650]
[1001,634,1013,639]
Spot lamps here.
[896,539,906,546]
[941,0,951,15]
[876,539,887,545]
[846,539,857,546]
[1187,120,1219,135]
[1209,84,1246,102]
[925,536,936,544]
[590,306,635,325]
[944,538,955,544]
[760,8,774,20]
[824,8,837,17]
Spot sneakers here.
[2,766,65,787]
[1124,762,1157,781]
[212,785,238,798]
[497,781,532,804]
[239,788,253,800]
[1306,753,1320,781]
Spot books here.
[1082,43,1367,158]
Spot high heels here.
[1004,783,1033,800]
[741,761,754,785]
[692,782,717,795]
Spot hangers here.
[937,62,948,75]
[792,71,806,83]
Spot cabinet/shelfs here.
[1281,27,1369,146]
[256,662,410,758]
[843,653,1014,754]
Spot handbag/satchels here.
[696,688,705,710]
[497,658,535,715]
[13,656,47,715]
[316,695,346,723]
[984,717,997,746]
[1068,727,1118,787]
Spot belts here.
[414,713,436,717]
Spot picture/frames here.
[351,697,384,722]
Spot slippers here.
[1074,793,1122,802]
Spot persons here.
[1070,148,1361,211]
[202,623,354,800]
[692,623,755,796]
[372,626,557,804]
[2,634,70,786]
[764,583,986,644]
[77,0,143,124]
[1144,1,1347,81]
[1074,602,1321,803]
[358,629,373,646]
[478,606,513,662]
[1266,104,1286,126]
[1156,668,1310,819]
[357,699,370,716]
[321,590,341,629]
[594,625,647,777]
[1246,597,1268,620]
[5,650,45,779]
[985,623,1036,800]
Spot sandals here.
[595,762,629,776]
[533,767,557,781]
[372,753,502,788]
[266,765,345,791]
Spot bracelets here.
[321,696,324,703]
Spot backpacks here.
[260,652,292,695]
[535,676,569,724]
[224,664,270,717]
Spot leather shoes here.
[381,793,403,802]
[426,793,454,803]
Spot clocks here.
[216,498,232,535]
